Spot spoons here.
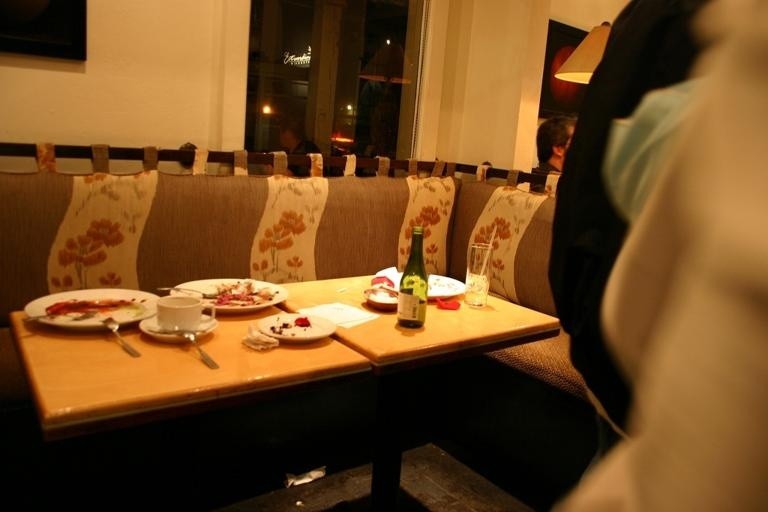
[176,330,219,370]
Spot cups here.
[465,242,494,308]
[155,296,216,333]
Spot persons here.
[546,0,767,440]
[272,112,321,156]
[531,116,576,177]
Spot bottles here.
[397,226,429,329]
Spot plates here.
[24,288,161,331]
[139,315,218,343]
[363,288,397,312]
[378,275,467,302]
[169,278,289,313]
[259,313,337,344]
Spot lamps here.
[554,21,613,85]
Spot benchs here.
[0,170,627,512]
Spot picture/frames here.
[538,18,590,119]
[0,0,88,63]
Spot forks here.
[99,317,141,359]
